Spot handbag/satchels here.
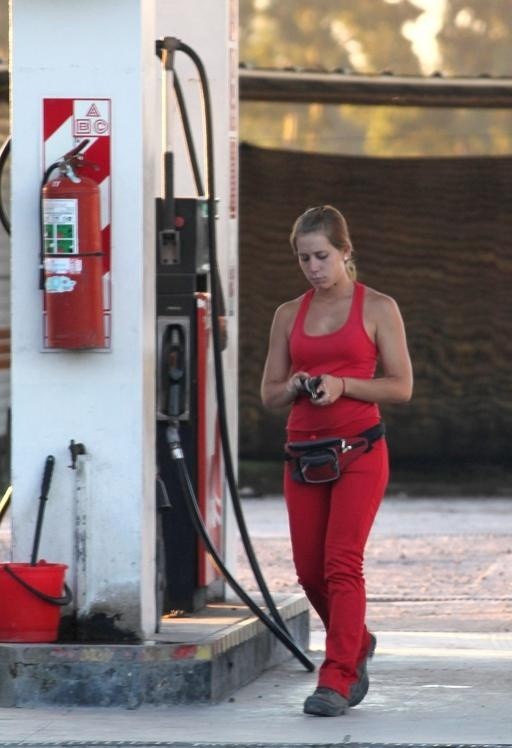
[283,423,388,484]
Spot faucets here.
[69,439,82,469]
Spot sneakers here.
[303,634,377,717]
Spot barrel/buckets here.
[0,561,72,643]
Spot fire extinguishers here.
[38,139,105,349]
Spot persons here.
[261,206,415,716]
